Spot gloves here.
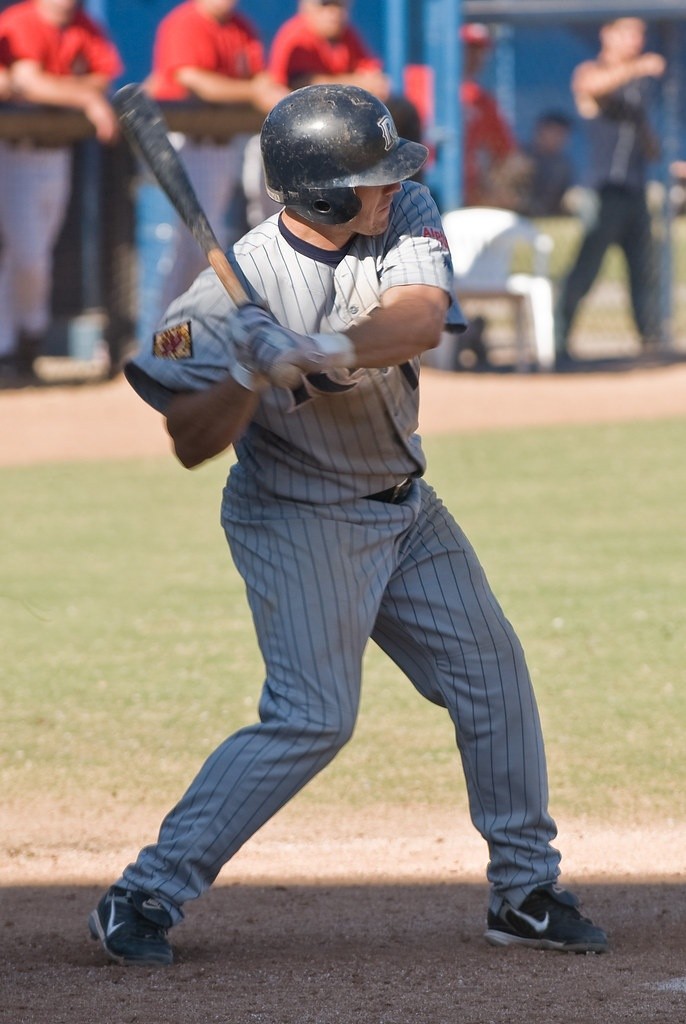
[227,305,284,393]
[247,323,358,381]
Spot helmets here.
[260,83,430,227]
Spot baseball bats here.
[107,81,305,390]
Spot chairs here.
[431,207,556,374]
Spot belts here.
[363,477,412,505]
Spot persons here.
[0,0,686,385]
[88,84,609,967]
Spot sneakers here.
[86,886,174,966]
[484,879,608,954]
